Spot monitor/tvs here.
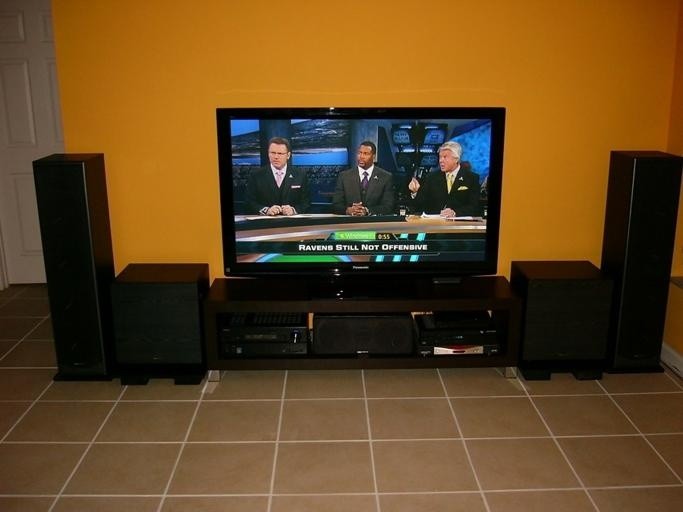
[290,119,349,166]
[390,122,448,168]
[231,119,262,166]
[216,107,507,297]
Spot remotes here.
[431,275,462,285]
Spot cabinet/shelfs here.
[204,277,521,382]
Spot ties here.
[275,170,284,188]
[447,173,455,194]
[360,171,370,193]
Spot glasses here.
[268,151,289,157]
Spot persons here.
[243,136,311,216]
[405,141,483,218]
[332,141,395,217]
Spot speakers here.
[312,313,416,357]
[32,152,116,382]
[600,149,683,374]
